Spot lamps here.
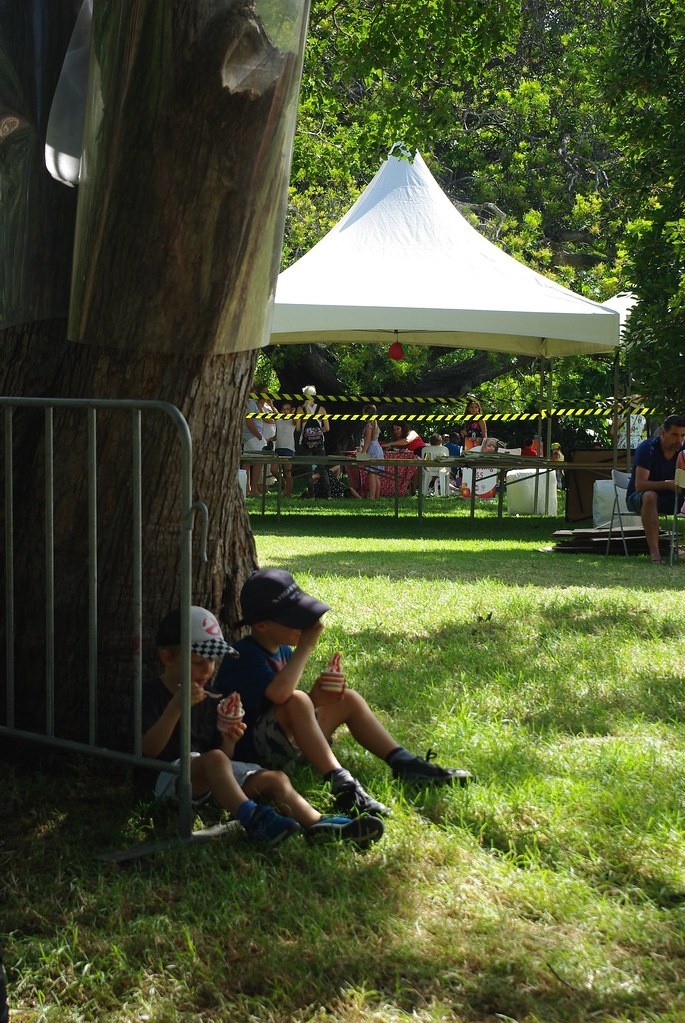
[387,333,405,362]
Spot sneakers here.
[303,814,384,851]
[242,805,301,848]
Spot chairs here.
[605,471,678,559]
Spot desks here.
[342,451,416,496]
[239,453,633,530]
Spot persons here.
[425,433,449,499]
[360,404,385,501]
[522,440,537,456]
[214,568,476,822]
[626,413,685,565]
[382,421,428,459]
[458,398,488,450]
[132,604,384,852]
[240,382,296,498]
[296,386,363,500]
[550,442,564,490]
[441,430,467,490]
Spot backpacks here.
[299,404,325,449]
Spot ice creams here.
[217,692,245,732]
[320,652,344,692]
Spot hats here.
[239,568,329,630]
[550,442,561,450]
[442,433,450,438]
[155,605,240,659]
[302,385,316,396]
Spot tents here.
[270,140,621,517]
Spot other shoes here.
[651,559,665,565]
[393,748,474,788]
[331,778,390,819]
